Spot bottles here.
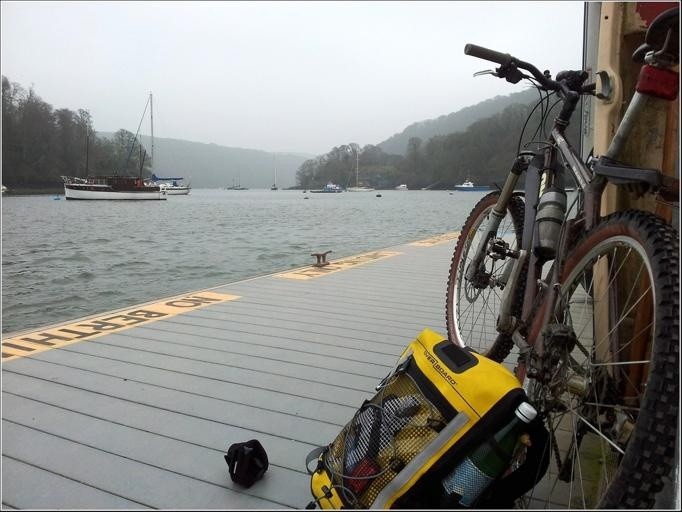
[441,401,537,510]
[533,187,567,260]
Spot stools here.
[311,249,332,267]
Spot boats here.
[59,95,192,200]
[454,181,490,191]
[395,184,408,191]
[346,148,375,192]
[227,177,248,190]
[310,185,342,193]
[271,167,278,190]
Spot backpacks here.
[305,328,552,509]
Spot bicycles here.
[444,5,680,507]
